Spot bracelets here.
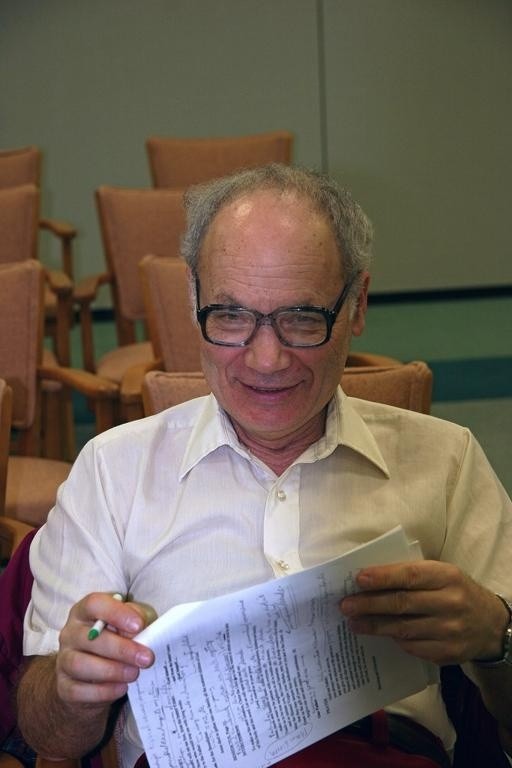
[480,594,512,675]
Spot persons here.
[13,161,511,767]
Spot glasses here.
[194,269,363,349]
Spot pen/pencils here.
[89,592,124,639]
